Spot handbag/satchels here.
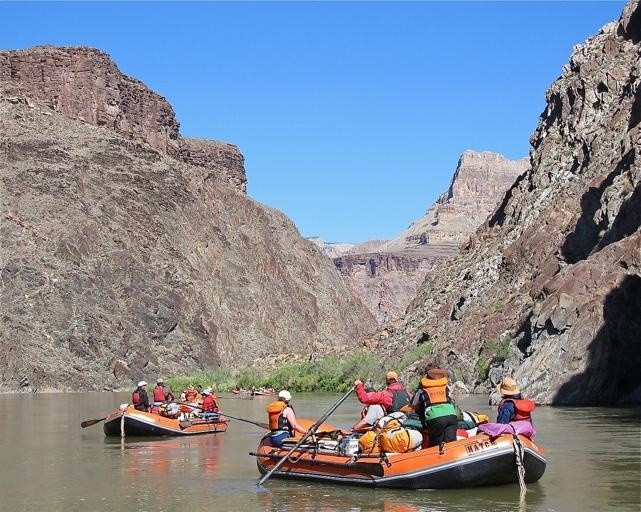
[358,402,488,453]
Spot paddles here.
[81,417,106,428]
[249,452,384,478]
[174,402,270,429]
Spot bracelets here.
[353,425,358,431]
[304,429,308,434]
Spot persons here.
[409,363,458,449]
[133,380,151,413]
[180,383,220,407]
[353,372,414,414]
[496,377,536,430]
[352,388,388,431]
[200,390,218,413]
[155,378,174,404]
[266,389,309,450]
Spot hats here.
[420,368,447,388]
[278,389,291,402]
[385,371,398,383]
[155,378,162,384]
[496,377,520,396]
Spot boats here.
[255,416,548,490]
[103,405,228,438]
[180,397,220,408]
[252,387,276,395]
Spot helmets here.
[137,380,147,388]
